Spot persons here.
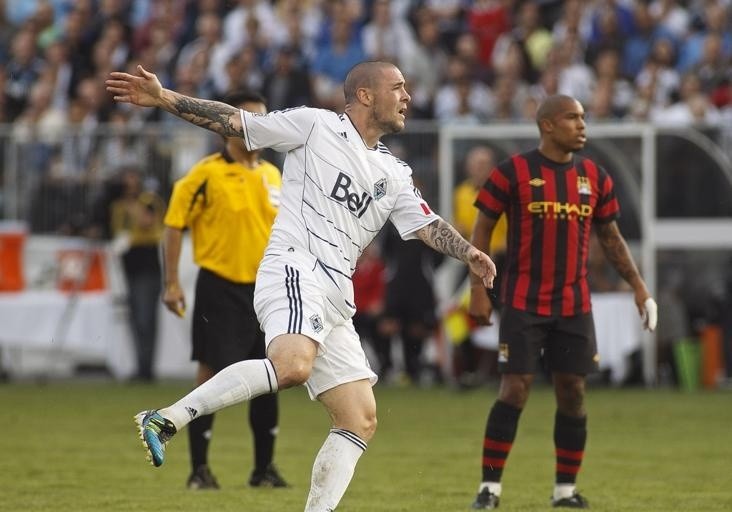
[161,88,296,491]
[461,94,658,508]
[104,59,498,510]
[1,2,731,390]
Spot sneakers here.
[549,489,589,508]
[133,408,177,467]
[250,461,286,487]
[471,487,499,509]
[187,463,220,490]
